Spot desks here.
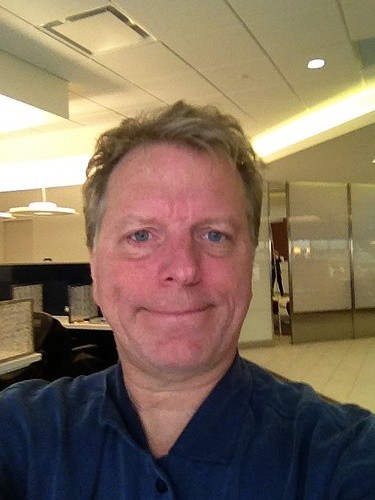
[2,278,118,396]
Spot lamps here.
[0,213,18,223]
[10,185,78,220]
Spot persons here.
[0,96,375,500]
[270,248,285,297]
